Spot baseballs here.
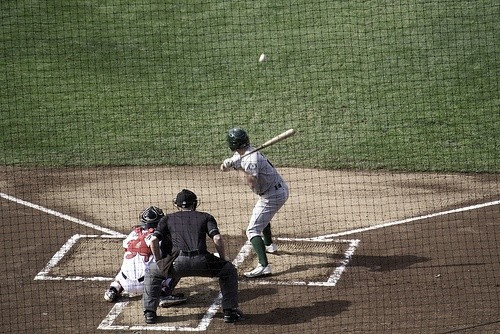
[258,54,267,63]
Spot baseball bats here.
[241,128,296,158]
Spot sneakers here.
[104,289,116,302]
[159,294,188,307]
[144,311,157,324]
[243,262,272,278]
[265,242,277,253]
[224,309,242,322]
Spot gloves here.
[231,152,241,169]
[223,158,234,169]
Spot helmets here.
[138,206,165,225]
[226,128,250,151]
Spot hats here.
[175,189,197,211]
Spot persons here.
[143,189,243,323]
[104,206,187,307]
[220,127,289,278]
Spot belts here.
[179,248,202,256]
[259,184,282,196]
[122,272,144,282]
